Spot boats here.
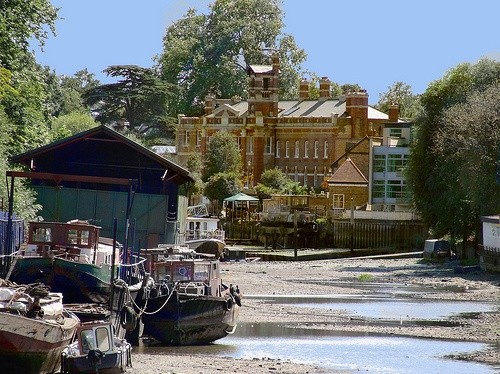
[1,218,242,373]
